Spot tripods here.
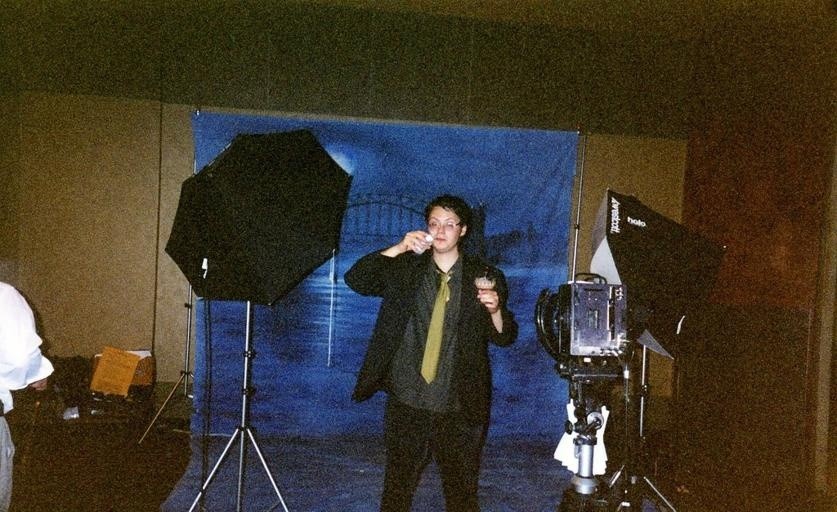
[136,283,197,450]
[608,355,678,512]
[188,301,294,511]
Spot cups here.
[412,230,437,255]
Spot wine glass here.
[474,263,497,305]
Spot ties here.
[417,269,455,385]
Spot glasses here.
[428,218,463,231]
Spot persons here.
[343,195,516,512]
[0,281,55,512]
